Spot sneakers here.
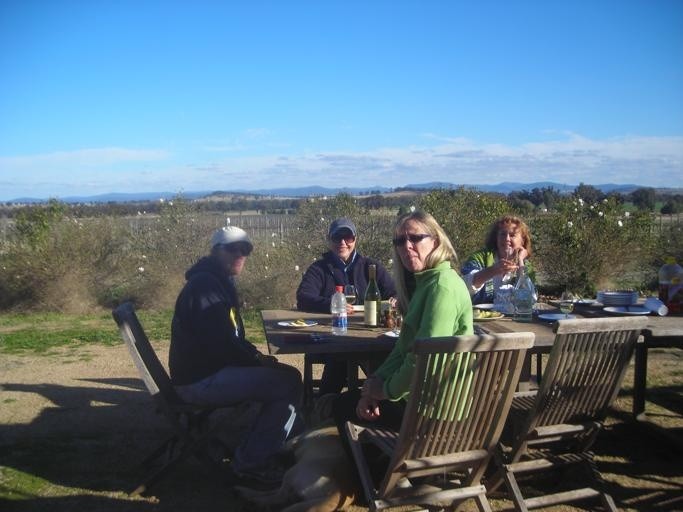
[232,473,278,496]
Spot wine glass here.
[344,285,358,305]
[560,292,573,320]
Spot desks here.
[260,305,682,427]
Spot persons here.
[168,225,301,485]
[294,217,400,393]
[328,212,474,480]
[462,214,544,304]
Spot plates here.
[602,306,651,316]
[574,298,595,304]
[537,313,575,321]
[472,304,493,310]
[596,290,639,306]
[471,314,504,322]
[277,320,318,327]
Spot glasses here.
[331,233,355,243]
[217,243,250,256]
[394,234,430,245]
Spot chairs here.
[111,301,249,496]
[334,331,535,512]
[484,314,649,512]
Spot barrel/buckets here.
[657,255,683,304]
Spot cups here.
[644,296,668,316]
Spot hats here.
[210,226,253,251]
[328,218,356,239]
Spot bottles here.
[512,266,533,323]
[331,285,348,336]
[363,264,382,328]
